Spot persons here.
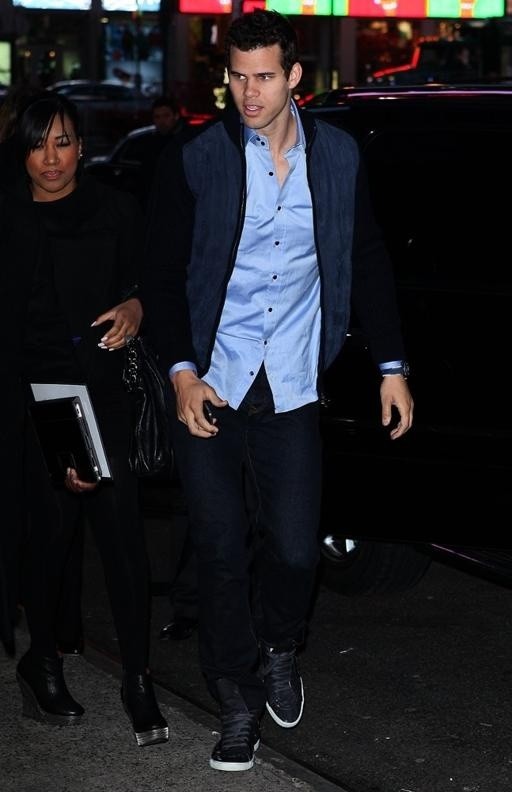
[150,94,176,125]
[1,89,186,748]
[434,41,477,84]
[140,11,420,772]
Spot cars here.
[47,79,156,162]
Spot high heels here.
[16,644,88,723]
[116,664,171,748]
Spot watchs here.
[379,360,412,382]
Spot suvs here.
[108,84,511,177]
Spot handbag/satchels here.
[105,333,174,481]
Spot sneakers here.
[208,705,265,773]
[257,638,305,731]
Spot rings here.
[125,333,134,345]
